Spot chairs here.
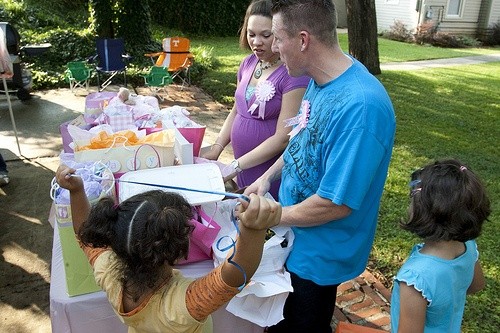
[139,66,172,102]
[87,39,132,93]
[144,37,195,91]
[64,61,96,96]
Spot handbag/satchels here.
[59,91,206,169]
[49,161,116,296]
[114,144,221,265]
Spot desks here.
[49,215,265,333]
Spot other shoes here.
[0,172,10,184]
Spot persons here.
[390,160,491,332]
[0,26,12,183]
[243,0,397,333]
[199,0,311,202]
[54,165,282,332]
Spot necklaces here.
[255,59,283,78]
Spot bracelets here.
[210,141,224,152]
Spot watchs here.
[231,159,242,177]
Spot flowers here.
[246,78,275,119]
[283,99,310,140]
